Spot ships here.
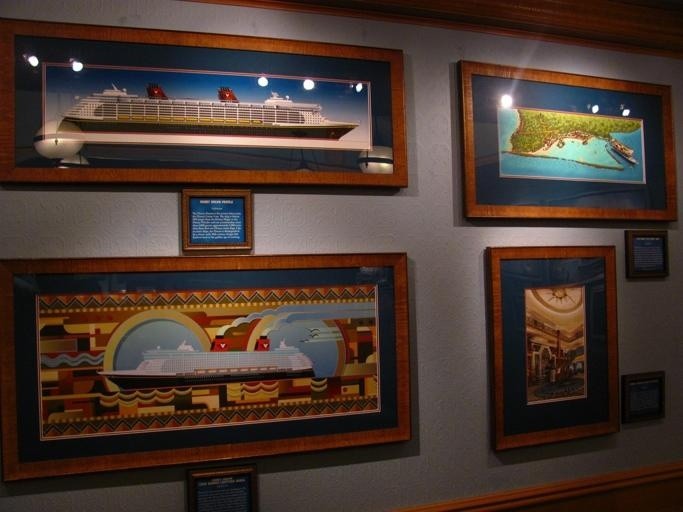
[60,81,362,144]
[95,334,320,390]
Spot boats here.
[610,138,640,168]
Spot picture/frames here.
[457,60,677,221]
[0,17,408,186]
[182,188,253,251]
[0,251,412,483]
[488,245,620,451]
[624,229,669,278]
[186,464,258,512]
[621,370,666,424]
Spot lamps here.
[620,104,631,118]
[69,57,83,72]
[586,102,600,114]
[349,81,364,94]
[21,52,39,68]
